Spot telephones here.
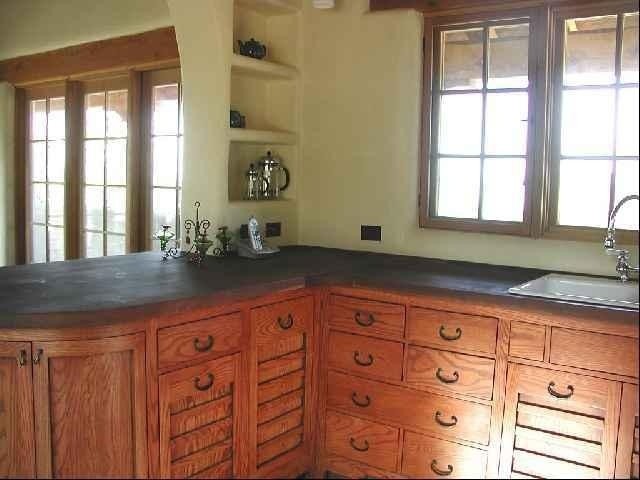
[236,215,281,259]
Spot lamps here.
[152,200,234,266]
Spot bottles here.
[243,149,291,201]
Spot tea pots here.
[237,37,267,60]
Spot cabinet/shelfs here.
[0,319,149,480]
[149,287,318,479]
[321,287,639,479]
[230,47,299,149]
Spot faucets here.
[603,194,640,249]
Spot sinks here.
[510,273,640,311]
